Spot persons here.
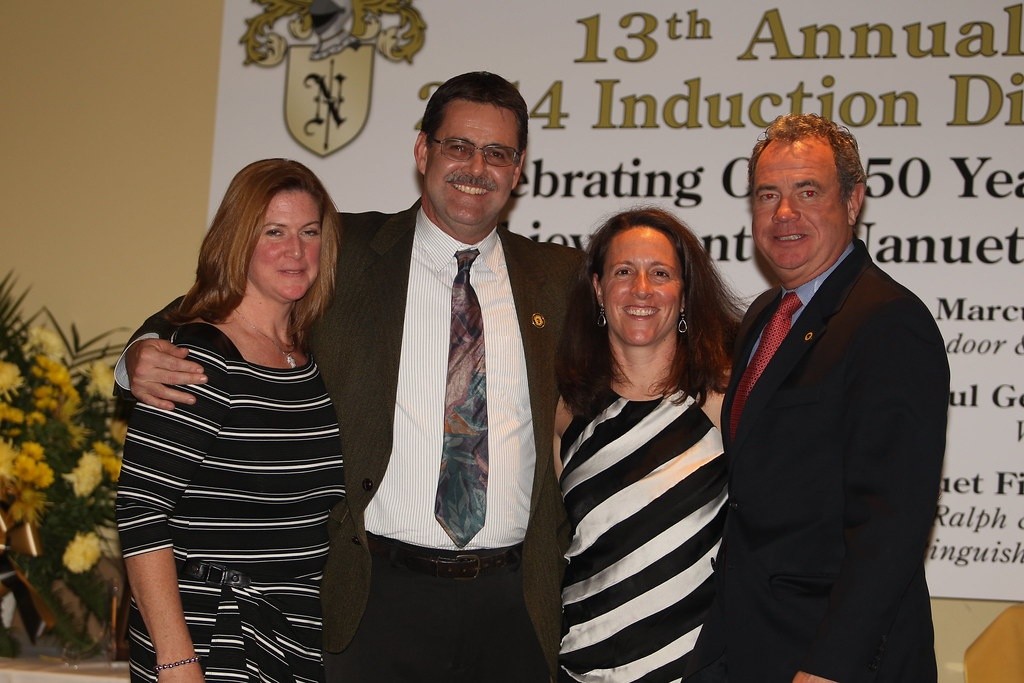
[679,112,951,683]
[113,158,347,683]
[553,209,745,683]
[113,70,585,683]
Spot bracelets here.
[155,655,198,671]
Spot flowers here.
[0,269,152,664]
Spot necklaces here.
[234,308,296,368]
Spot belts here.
[366,537,522,580]
[176,561,325,588]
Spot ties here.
[434,249,488,549]
[729,291,805,444]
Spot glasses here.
[430,137,520,167]
